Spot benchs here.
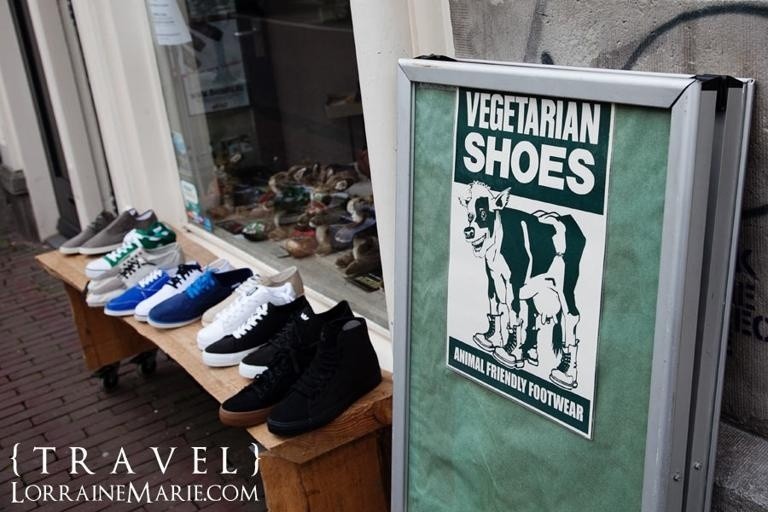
[36,223,393,512]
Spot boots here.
[474,313,503,352]
[494,322,524,368]
[550,345,579,390]
[521,326,539,366]
